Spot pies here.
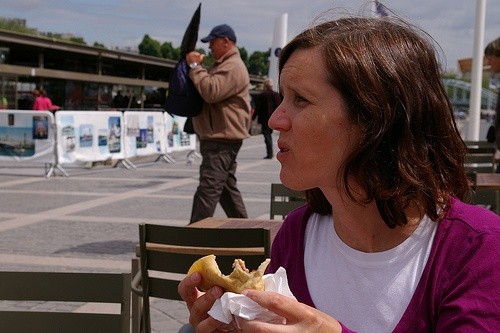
[188,255,271,294]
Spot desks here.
[182,216,285,247]
[474,171,500,196]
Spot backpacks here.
[166,60,204,117]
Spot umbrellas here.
[164,2,202,134]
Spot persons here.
[251,78,282,159]
[484,35,500,173]
[35,123,47,138]
[32,91,59,111]
[177,18,500,333]
[186,23,252,225]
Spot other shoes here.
[263,156,272,159]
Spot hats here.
[201,24,237,43]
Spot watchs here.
[189,63,201,68]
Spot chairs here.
[464,140,500,215]
[138,221,285,333]
[270,183,307,220]
